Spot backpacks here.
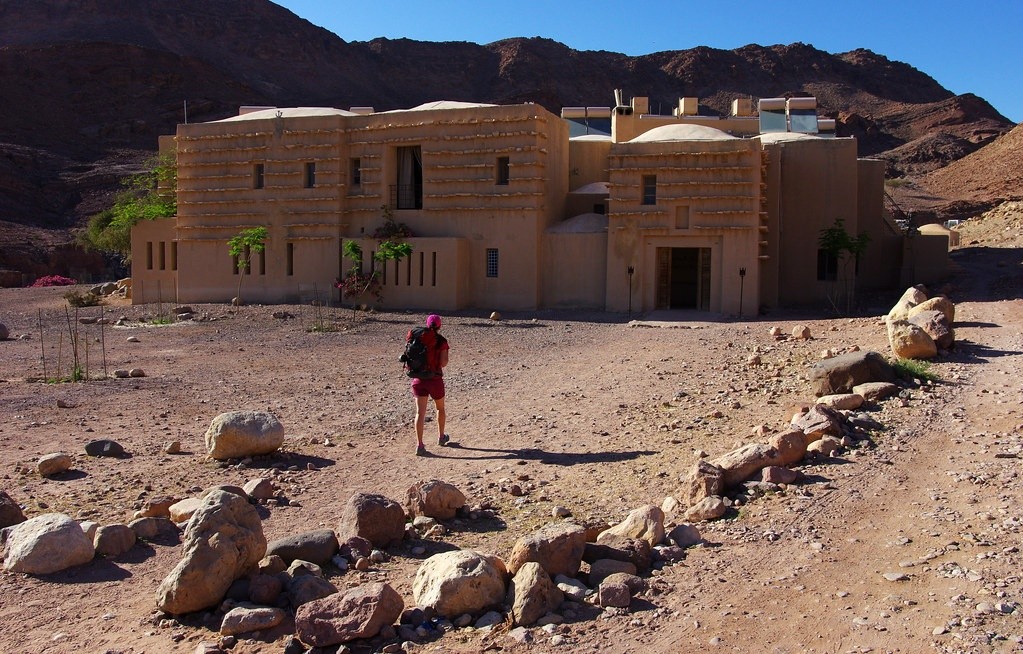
[403,327,430,379]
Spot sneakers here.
[438,434,449,446]
[416,446,427,455]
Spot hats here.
[426,314,442,328]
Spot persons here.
[406,314,450,456]
